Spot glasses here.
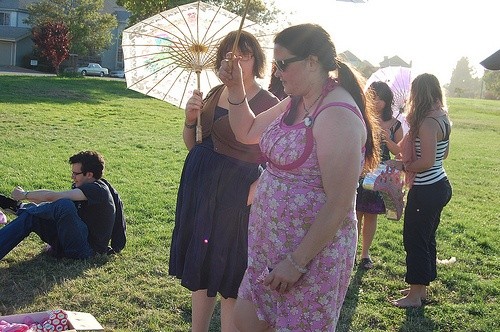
[275,57,305,72]
[224,52,255,61]
[71,171,83,176]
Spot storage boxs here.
[0,309,105,332]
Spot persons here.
[0,151,127,263]
[351,81,403,268]
[168,29,280,332]
[384,72,452,308]
[219,23,382,332]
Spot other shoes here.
[361,257,375,269]
[0,193,23,215]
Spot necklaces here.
[25,190,30,200]
[302,93,323,121]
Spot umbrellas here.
[119,1,273,144]
[480,50,500,70]
[363,66,423,139]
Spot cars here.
[75,62,109,78]
[110,69,125,78]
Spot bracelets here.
[185,117,198,129]
[286,252,308,274]
[402,162,407,172]
[227,94,247,106]
